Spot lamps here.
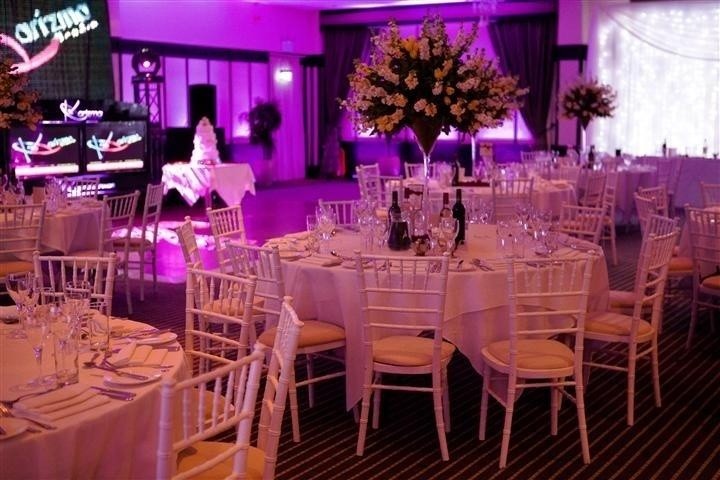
[279,67,294,84]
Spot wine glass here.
[421,148,633,191]
[0,272,179,439]
[266,186,564,272]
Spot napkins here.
[489,258,523,270]
[115,343,167,368]
[553,246,582,258]
[298,252,343,267]
[14,383,111,419]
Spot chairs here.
[353,248,457,463]
[33,256,113,317]
[683,202,720,356]
[207,201,249,274]
[0,173,165,314]
[179,296,304,480]
[176,218,263,381]
[606,211,679,335]
[558,226,680,428]
[318,145,720,278]
[223,238,360,442]
[185,262,257,443]
[478,252,594,468]
[158,342,265,479]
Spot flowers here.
[0,47,42,141]
[332,8,527,153]
[557,70,619,131]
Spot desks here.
[161,161,256,234]
[1,306,188,480]
[263,221,608,411]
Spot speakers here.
[189,83,217,126]
